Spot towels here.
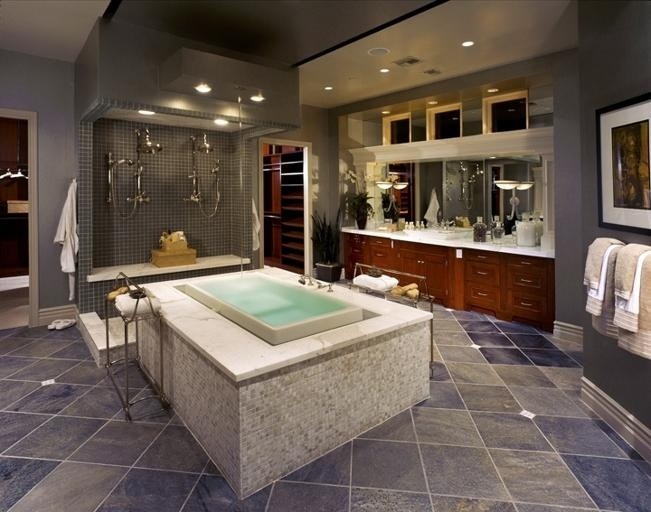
[107,285,163,318]
[582,236,651,361]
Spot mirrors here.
[374,156,543,231]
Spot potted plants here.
[310,210,341,283]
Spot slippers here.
[56,319,76,331]
[47,319,63,330]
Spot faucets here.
[298,273,313,285]
[317,279,327,289]
[445,218,456,230]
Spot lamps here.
[514,181,535,221]
[376,181,393,213]
[392,181,409,223]
[492,180,520,220]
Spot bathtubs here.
[184,271,363,346]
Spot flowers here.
[341,169,375,219]
[375,175,400,208]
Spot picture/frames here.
[595,91,651,236]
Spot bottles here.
[473,217,486,242]
[404,221,425,231]
[516,213,536,247]
[490,216,503,239]
[531,212,544,245]
[492,223,506,244]
[511,221,520,243]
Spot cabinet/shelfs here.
[343,233,555,332]
[262,143,304,269]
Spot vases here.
[383,201,395,224]
[355,207,368,229]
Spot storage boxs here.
[151,248,196,268]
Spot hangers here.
[0,167,28,180]
